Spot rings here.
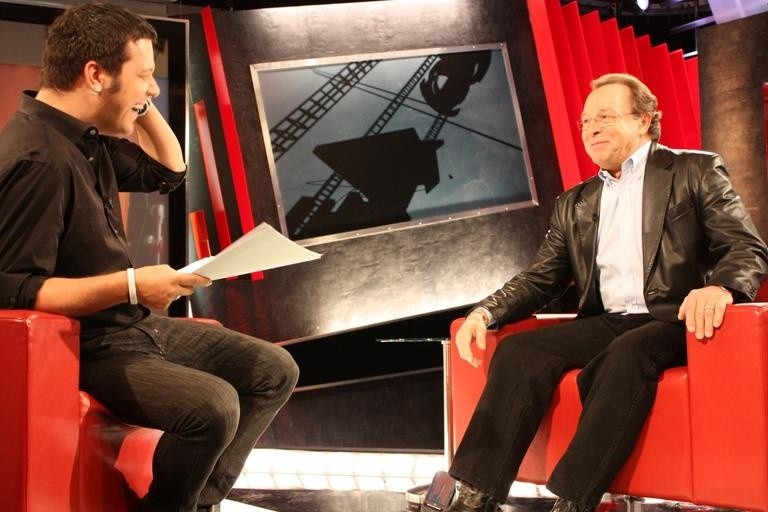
[703,305,715,309]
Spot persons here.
[447,73,768,512]
[0,0,300,512]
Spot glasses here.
[576,110,641,130]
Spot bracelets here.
[127,267,138,306]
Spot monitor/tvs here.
[247,42,542,248]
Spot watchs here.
[139,98,152,117]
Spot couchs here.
[0,310,225,511]
[451,303,768,512]
